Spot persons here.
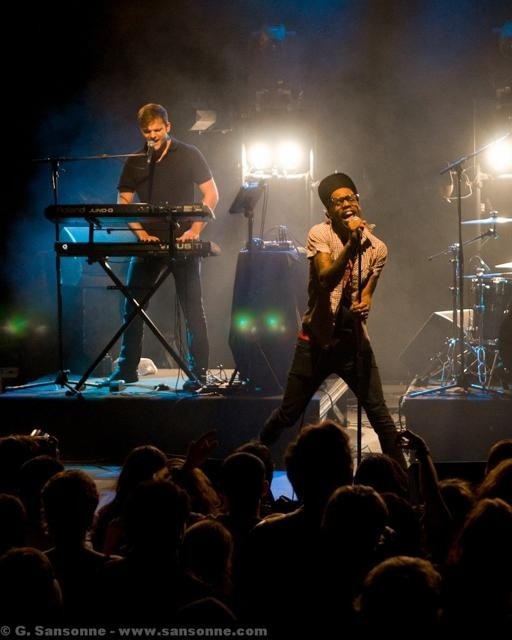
[104,103,219,390]
[259,171,407,473]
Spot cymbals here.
[494,262,511,271]
[462,217,511,224]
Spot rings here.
[361,312,364,316]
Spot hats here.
[317,172,360,212]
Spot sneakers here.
[182,373,207,392]
[95,367,139,387]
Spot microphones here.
[146,140,154,164]
[349,216,363,243]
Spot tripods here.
[221,254,283,394]
[407,134,512,397]
[4,152,104,397]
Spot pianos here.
[45,203,221,257]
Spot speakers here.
[396,308,474,382]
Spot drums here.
[469,276,512,340]
